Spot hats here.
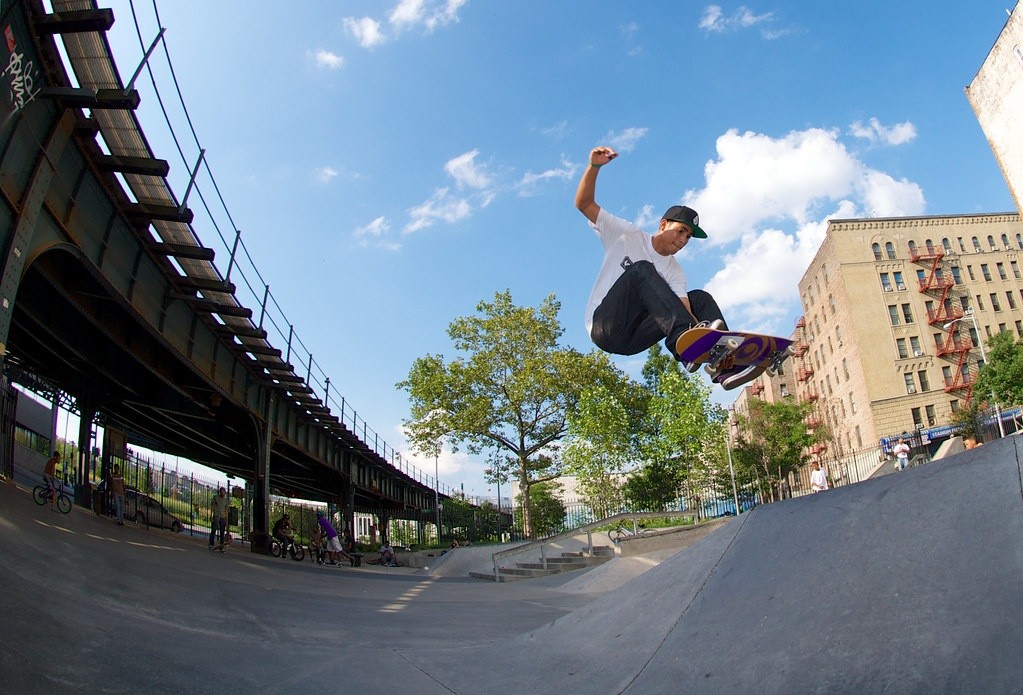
[662,205,707,238]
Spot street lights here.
[942,315,1006,438]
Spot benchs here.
[323,550,365,567]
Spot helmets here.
[283,514,290,518]
[316,512,323,516]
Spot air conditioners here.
[808,335,813,341]
[965,310,971,314]
[837,341,842,347]
[781,391,789,397]
[898,285,905,290]
[908,388,915,394]
[975,248,983,253]
[991,246,999,251]
[885,286,892,292]
[946,249,954,255]
[1005,245,1013,250]
[915,423,923,430]
[914,350,922,357]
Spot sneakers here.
[717,364,767,389]
[681,319,724,373]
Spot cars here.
[106,488,185,533]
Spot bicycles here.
[366,549,404,567]
[311,537,325,565]
[33,476,73,514]
[268,531,305,561]
[608,519,658,543]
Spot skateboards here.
[320,563,341,568]
[211,540,232,552]
[675,327,799,378]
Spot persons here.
[451,538,460,549]
[208,487,230,554]
[315,511,356,567]
[336,528,359,568]
[380,540,395,566]
[810,461,828,493]
[463,539,470,546]
[72,467,77,488]
[307,524,325,566]
[893,435,910,472]
[108,463,127,526]
[43,451,61,514]
[272,515,298,560]
[70,477,74,487]
[574,146,767,392]
[64,467,69,487]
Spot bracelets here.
[590,162,602,168]
